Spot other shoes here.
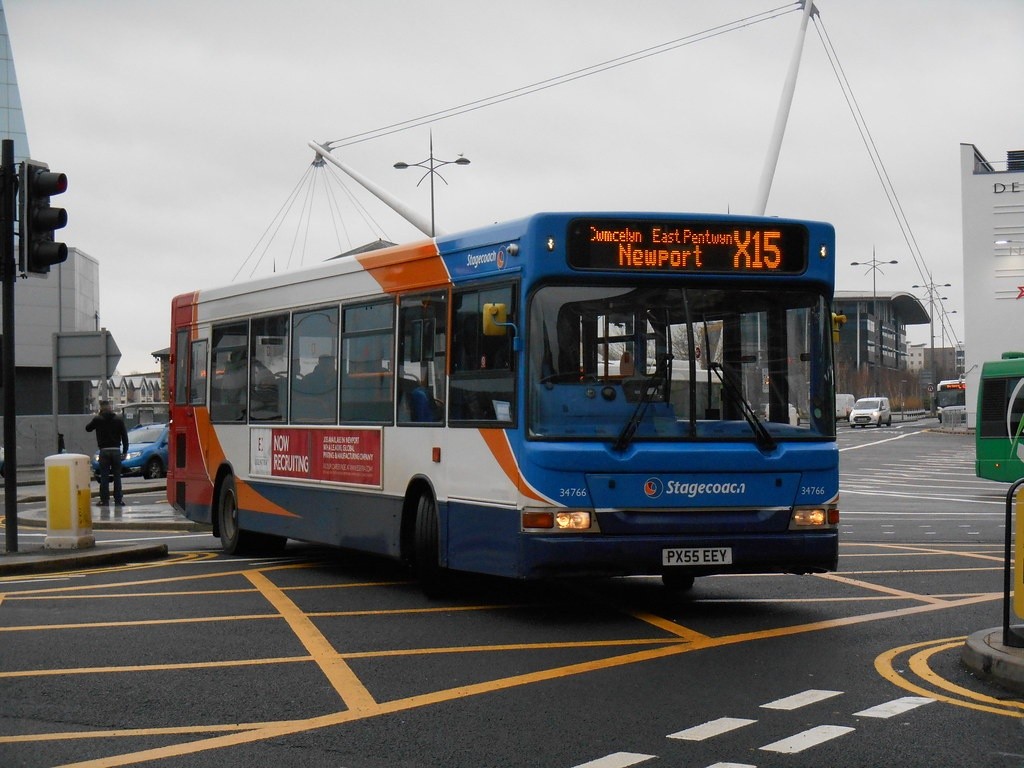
[96,500,109,506]
[115,501,125,506]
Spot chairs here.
[273,370,334,421]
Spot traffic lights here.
[13,156,68,280]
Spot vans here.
[850,397,892,428]
[834,393,855,422]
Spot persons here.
[222,352,257,405]
[302,354,338,391]
[86,400,130,507]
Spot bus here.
[973,350,1024,483]
[936,379,966,424]
[163,136,849,605]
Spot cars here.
[90,424,171,486]
[765,402,801,426]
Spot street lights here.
[911,281,957,407]
[848,243,900,350]
[390,127,471,242]
